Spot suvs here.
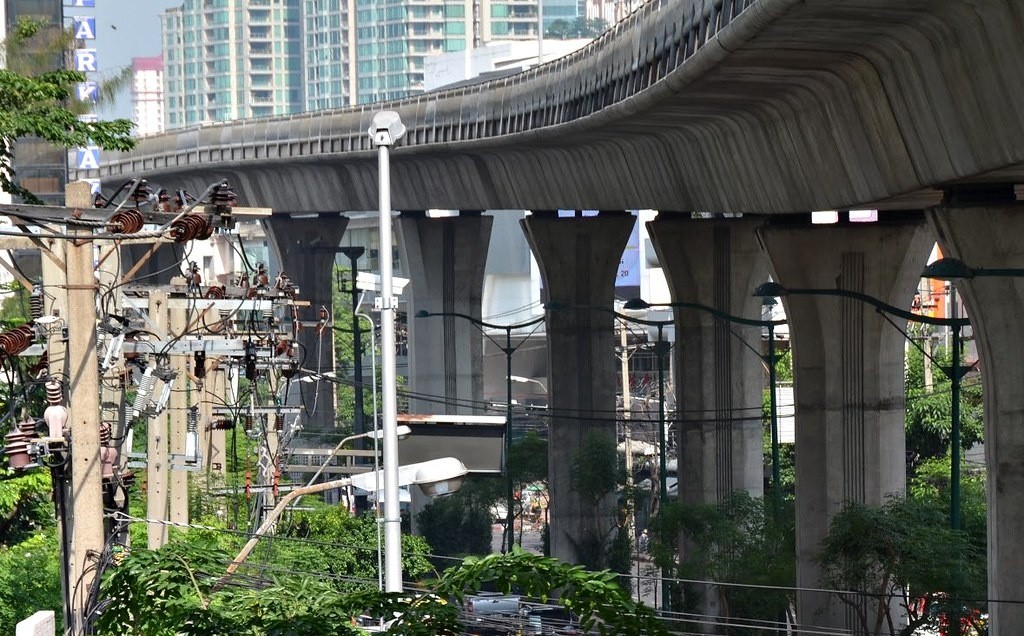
[507,604,580,636]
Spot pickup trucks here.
[455,594,523,616]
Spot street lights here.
[749,281,971,636]
[330,246,408,572]
[413,308,545,556]
[367,109,408,631]
[622,297,793,636]
